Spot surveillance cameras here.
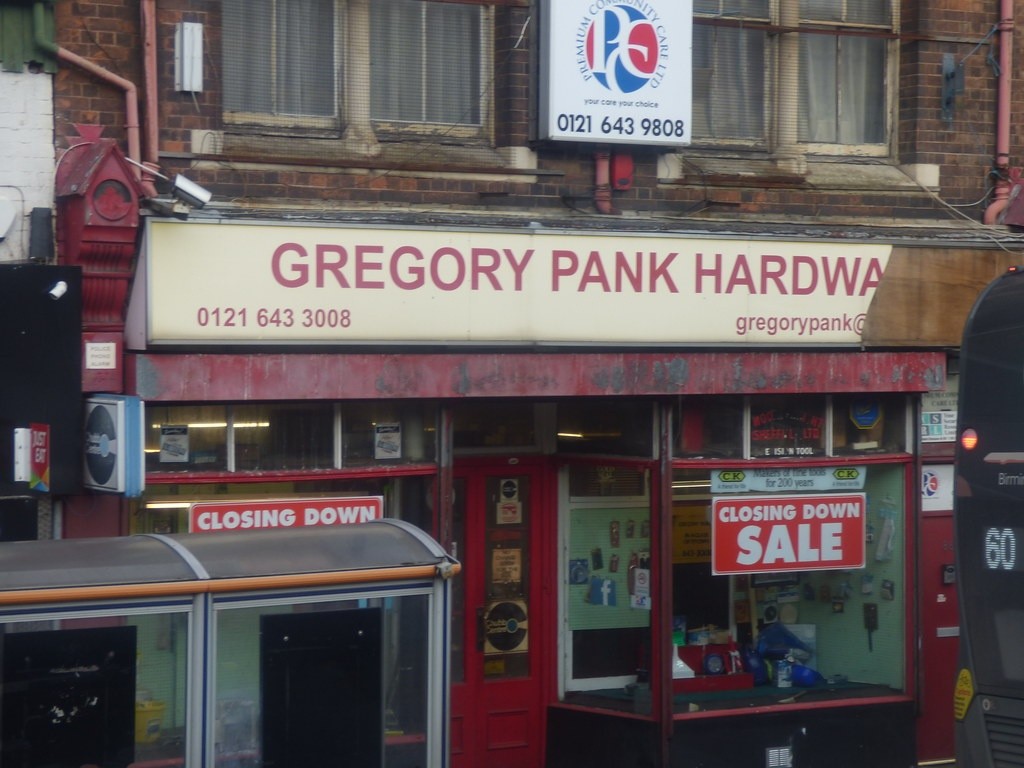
[171,173,213,211]
